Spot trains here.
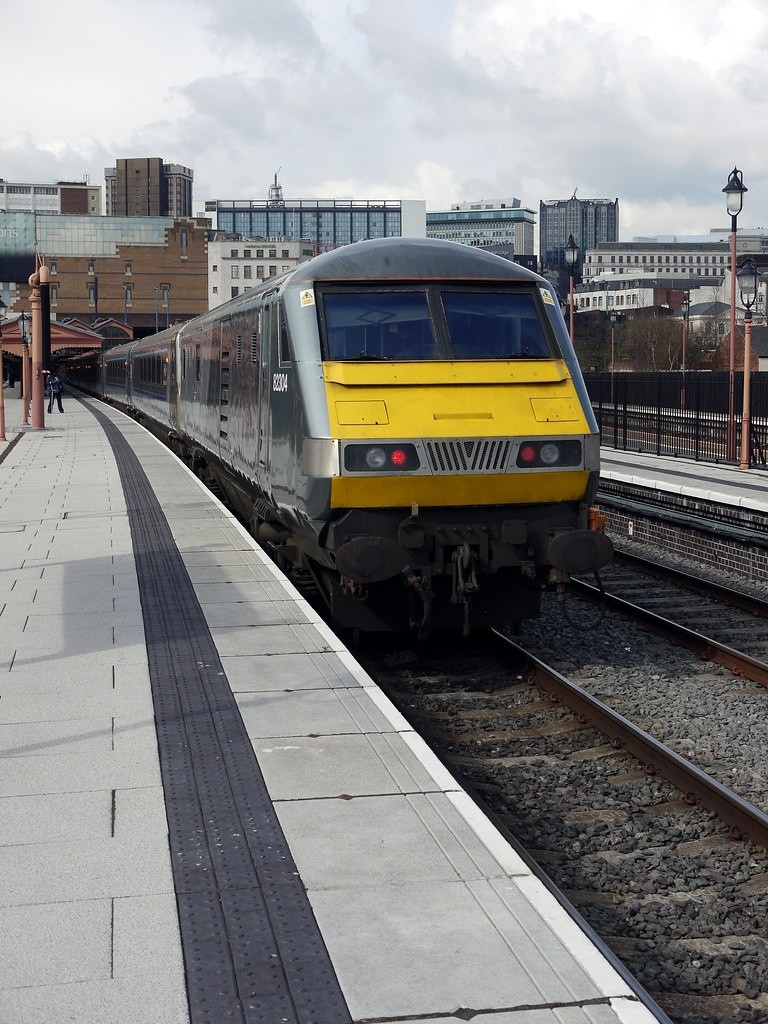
[64,236,614,632]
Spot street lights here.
[18,307,32,424]
[563,233,580,341]
[680,296,690,409]
[735,256,763,471]
[722,166,749,463]
[0,294,7,441]
[607,308,619,404]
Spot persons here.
[46,369,65,413]
